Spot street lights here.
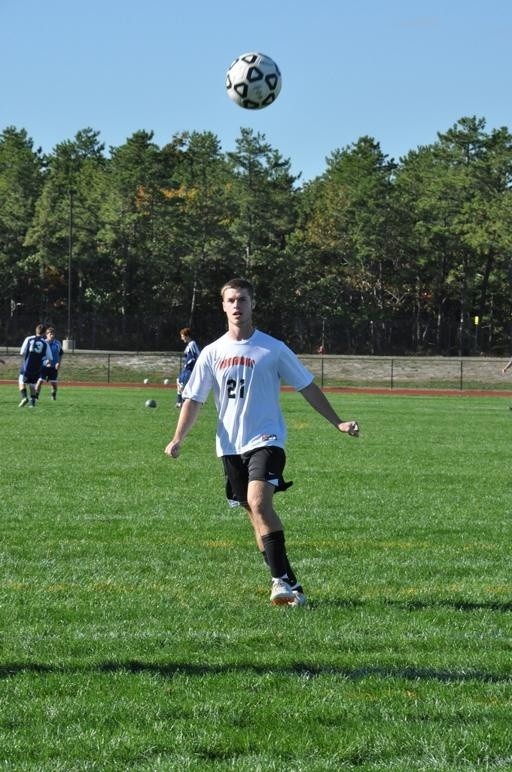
[474,315,479,356]
[62,189,78,350]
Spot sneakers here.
[270,580,307,608]
[18,390,55,408]
[176,403,182,407]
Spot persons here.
[175,328,200,408]
[34,327,64,401]
[18,324,53,408]
[503,362,512,410]
[164,279,360,606]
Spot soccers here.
[143,379,150,384]
[145,399,157,408]
[227,53,281,109]
[164,379,170,385]
